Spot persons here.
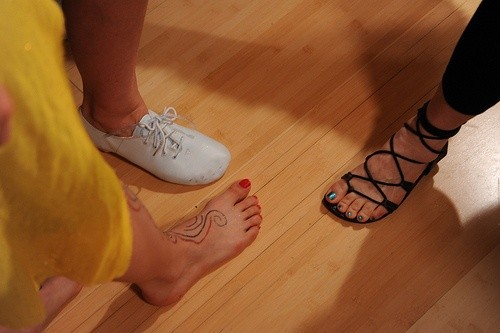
[0,0,263,332]
[323,0,500,224]
[60,0,230,185]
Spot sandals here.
[321,99,466,223]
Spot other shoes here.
[78,104,231,185]
